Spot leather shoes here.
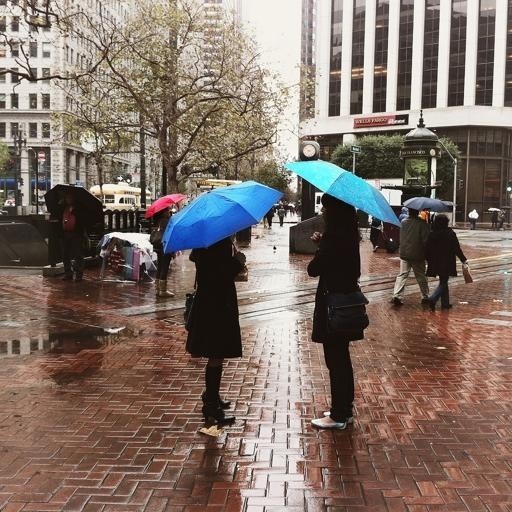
[389,297,404,306]
[441,303,452,309]
[322,410,354,425]
[420,298,429,304]
[202,406,236,423]
[424,298,435,313]
[201,391,231,407]
[310,416,347,431]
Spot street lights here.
[429,139,459,229]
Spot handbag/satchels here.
[324,287,370,336]
[183,291,197,330]
[462,261,473,284]
[234,265,248,282]
[149,227,164,246]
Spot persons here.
[399,206,408,223]
[426,213,468,312]
[391,208,430,307]
[369,215,381,252]
[150,207,177,299]
[307,192,368,429]
[498,208,506,231]
[54,190,90,282]
[491,210,498,229]
[468,208,479,230]
[185,233,246,426]
[260,194,303,230]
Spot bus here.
[88,183,151,211]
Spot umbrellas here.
[43,183,104,227]
[143,194,186,220]
[160,181,284,256]
[283,160,403,239]
[402,196,455,221]
[488,207,500,211]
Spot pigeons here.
[254,236,259,240]
[272,245,277,251]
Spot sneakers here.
[75,271,83,281]
[63,275,73,281]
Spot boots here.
[154,279,176,298]
